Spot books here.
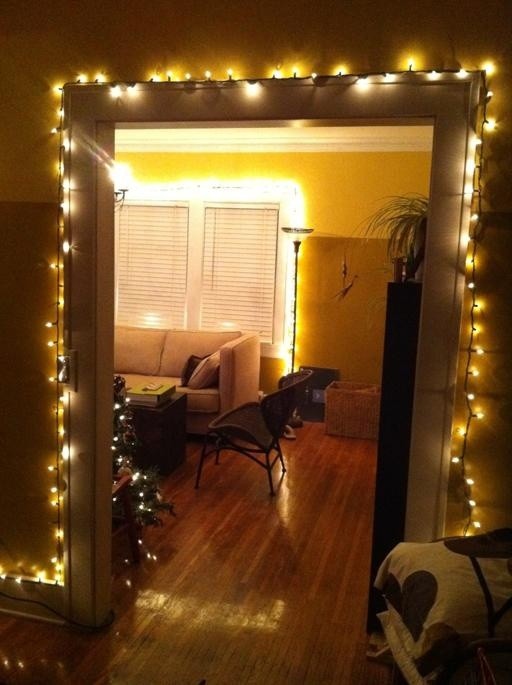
[126,383,176,409]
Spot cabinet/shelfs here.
[111,475,140,564]
[364,280,424,636]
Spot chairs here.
[194,370,314,495]
[443,526,512,685]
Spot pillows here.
[176,349,222,390]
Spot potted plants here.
[326,192,430,302]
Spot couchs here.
[114,326,261,441]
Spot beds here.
[364,541,511,685]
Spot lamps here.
[281,226,314,427]
